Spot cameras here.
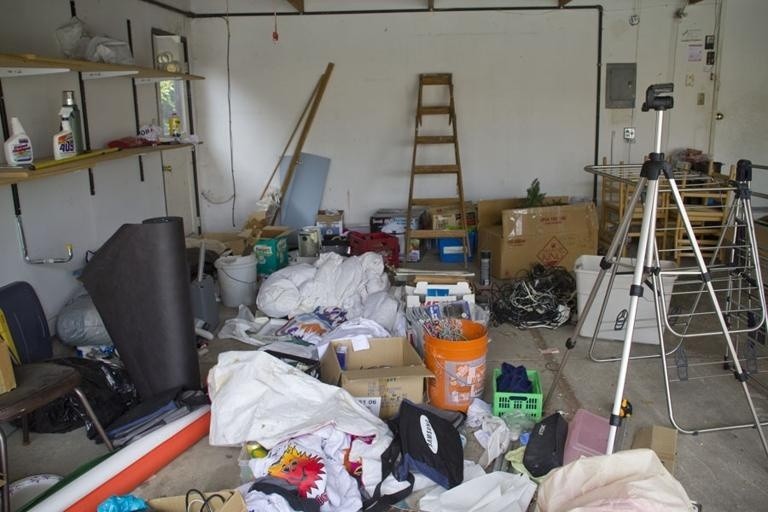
[642,82,676,110]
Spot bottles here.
[167,110,182,139]
[479,250,491,285]
[61,91,84,155]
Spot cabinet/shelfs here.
[0,53,206,187]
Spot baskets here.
[491,366,544,426]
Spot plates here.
[7,473,64,511]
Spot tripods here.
[723,181,755,373]
[543,111,768,454]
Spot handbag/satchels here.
[385,397,469,491]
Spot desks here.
[583,163,737,258]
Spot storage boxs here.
[243,222,300,277]
[317,333,438,421]
[316,208,344,243]
[747,215,768,291]
[561,406,622,469]
[396,279,488,360]
[370,200,477,266]
[571,253,682,347]
[476,193,599,283]
[190,231,248,259]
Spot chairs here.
[0,306,117,510]
[598,154,738,268]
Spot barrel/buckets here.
[422,318,491,415]
[214,255,259,309]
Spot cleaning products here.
[2,117,34,168]
[52,106,77,160]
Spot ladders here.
[405,73,473,257]
